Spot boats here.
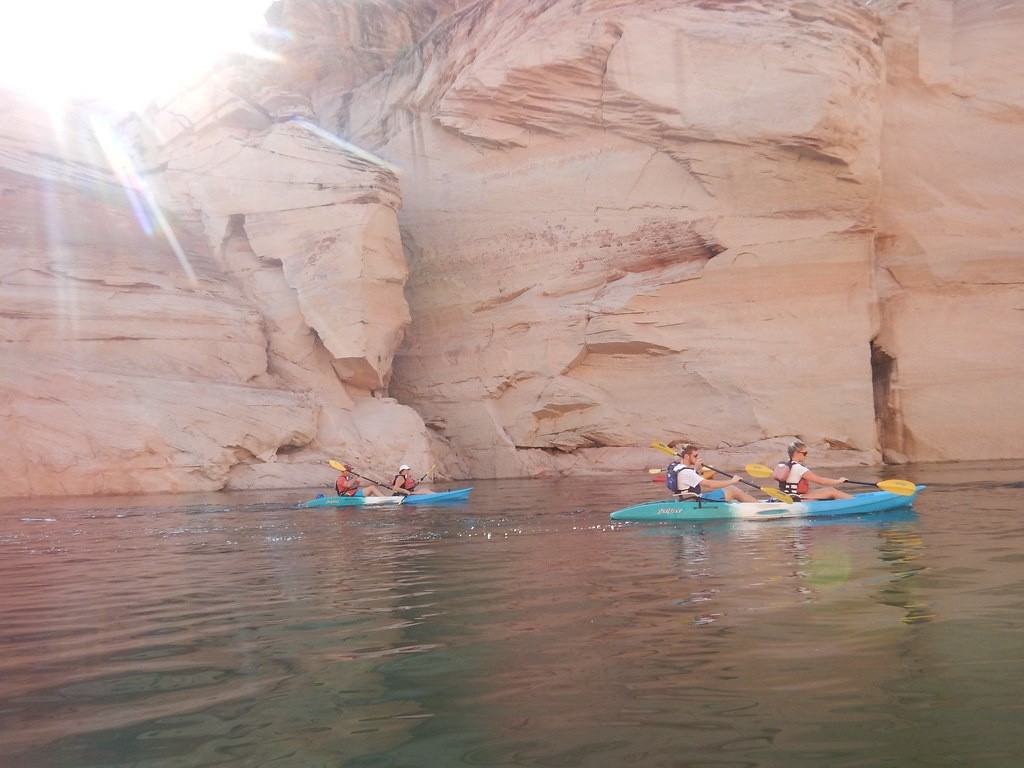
[651,469,718,487]
[297,487,474,509]
[609,484,926,522]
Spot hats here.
[343,465,354,471]
[399,465,411,472]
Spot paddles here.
[398,464,438,505]
[743,464,916,497]
[650,441,795,504]
[328,458,395,491]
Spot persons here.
[780,441,855,503]
[392,465,437,494]
[335,464,386,497]
[666,445,762,503]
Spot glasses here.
[691,453,698,458]
[797,451,807,456]
